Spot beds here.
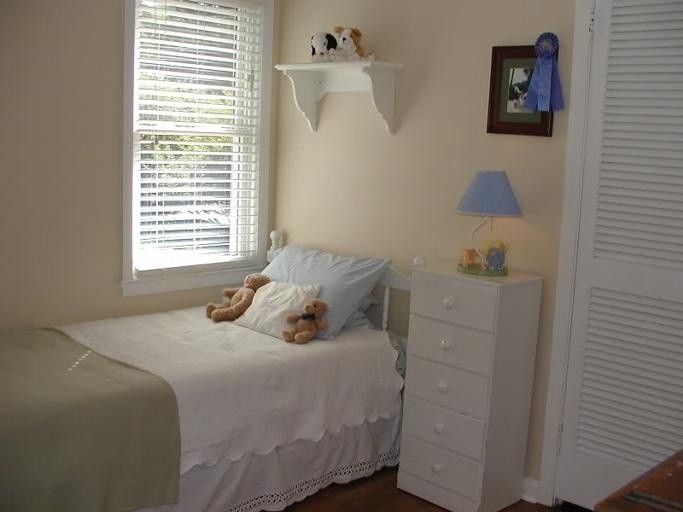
[0,230,425,512]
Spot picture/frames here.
[487,45,553,137]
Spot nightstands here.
[396,263,544,512]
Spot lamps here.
[454,170,521,275]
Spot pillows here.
[234,282,320,340]
[261,244,392,339]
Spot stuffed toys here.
[283,299,330,343]
[330,25,375,63]
[205,272,269,323]
[308,29,335,61]
